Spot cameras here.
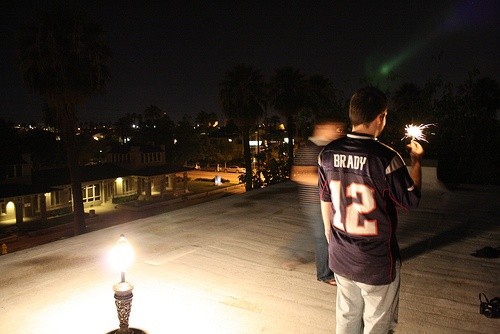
[480,298,500,318]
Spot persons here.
[316,85,427,334]
[289,118,346,287]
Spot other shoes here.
[320,278,337,286]
